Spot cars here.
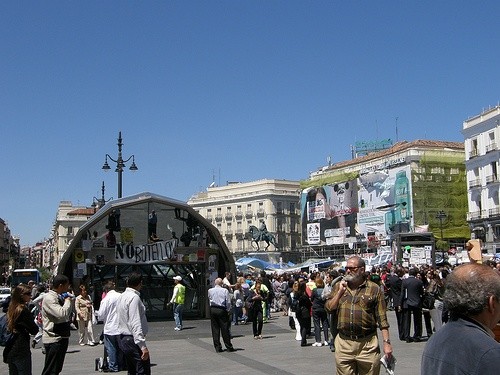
[0,287,11,302]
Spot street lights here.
[91,180,114,209]
[102,131,138,198]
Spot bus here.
[11,269,40,295]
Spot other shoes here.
[254,334,263,339]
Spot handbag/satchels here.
[422,295,435,309]
[54,322,71,337]
[2,333,19,363]
[137,359,151,375]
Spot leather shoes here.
[406,338,423,343]
[216,348,237,352]
[32,340,36,348]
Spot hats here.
[173,276,182,281]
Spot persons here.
[116,274,152,375]
[221,259,499,352]
[324,255,393,375]
[41,274,73,375]
[3,283,40,375]
[166,275,186,331]
[22,278,117,348]
[92,206,213,248]
[208,278,238,352]
[420,262,500,375]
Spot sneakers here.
[79,340,103,346]
[292,325,335,352]
[173,327,183,331]
[69,323,77,330]
[233,316,248,325]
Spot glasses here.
[345,267,361,270]
[19,292,31,296]
